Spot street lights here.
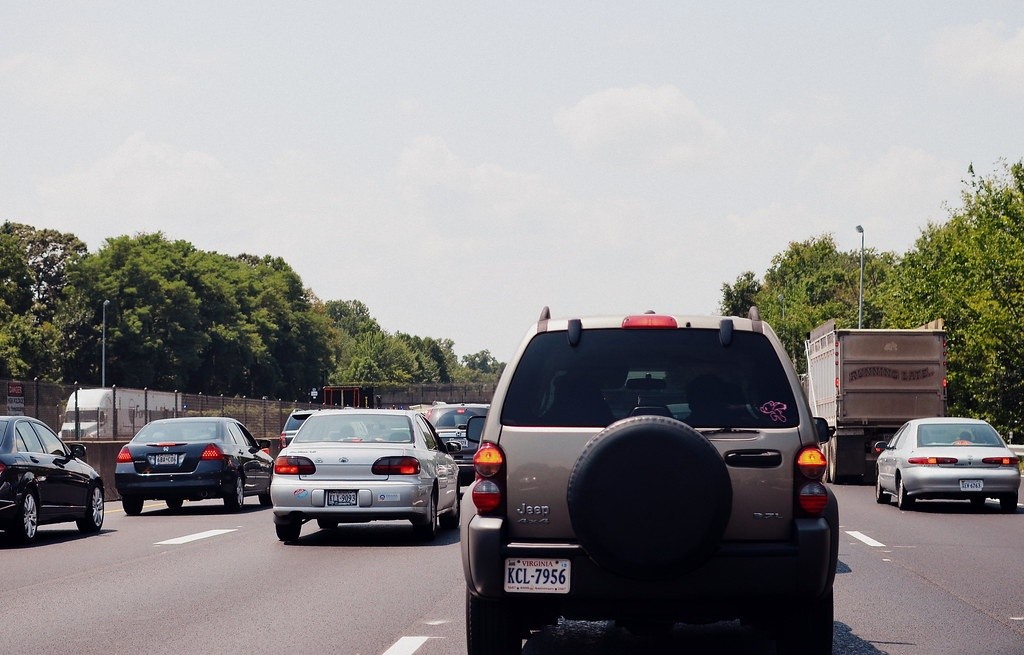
[102,300,110,386]
[856,225,864,328]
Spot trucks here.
[57,389,182,439]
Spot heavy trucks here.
[800,319,948,486]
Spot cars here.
[271,408,463,544]
[875,417,1022,515]
[115,417,275,516]
[0,415,104,547]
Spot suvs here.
[459,306,840,655]
[425,402,491,475]
[279,407,352,452]
